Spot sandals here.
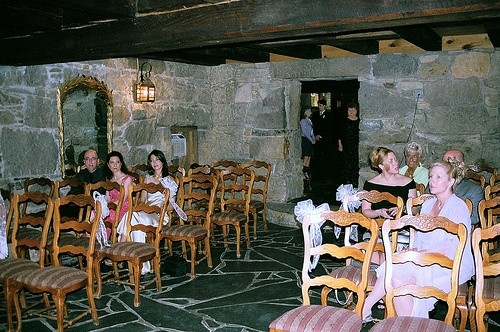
[377,300,385,310]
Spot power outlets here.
[415,89,423,100]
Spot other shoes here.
[104,259,122,268]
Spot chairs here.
[268,164,500,332]
[0,160,271,332]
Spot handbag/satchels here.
[93,192,109,219]
[164,253,187,276]
[328,265,377,306]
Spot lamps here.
[136,62,155,103]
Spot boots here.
[303,166,309,181]
[305,168,312,180]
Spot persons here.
[299,100,483,325]
[0,196,8,260]
[62,149,177,276]
